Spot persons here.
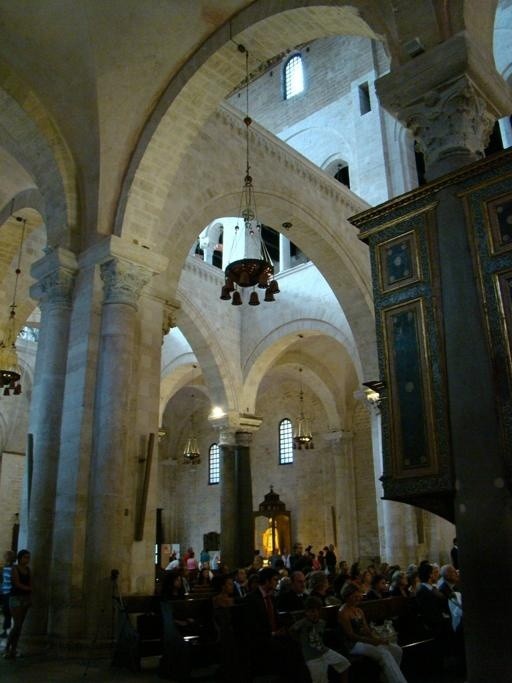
[1,548,15,638]
[5,549,35,656]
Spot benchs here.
[133,571,467,683]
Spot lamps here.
[362,381,388,408]
[292,334,315,450]
[221,49,282,305]
[0,219,28,396]
[184,366,201,465]
